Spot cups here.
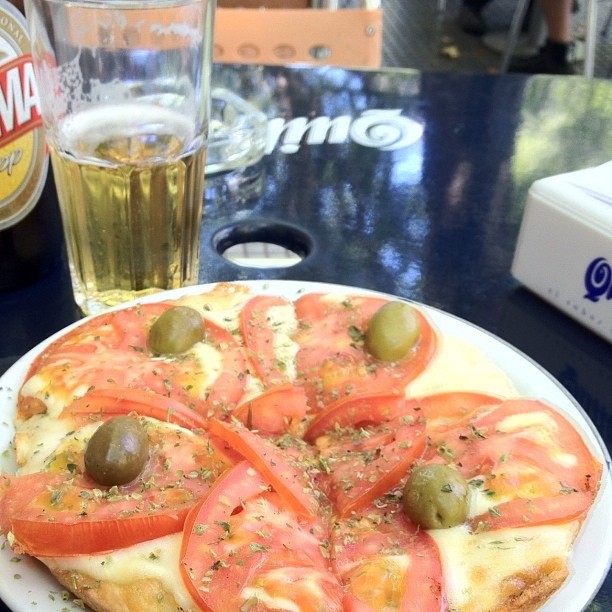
[23,0,218,315]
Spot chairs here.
[68,9,382,66]
